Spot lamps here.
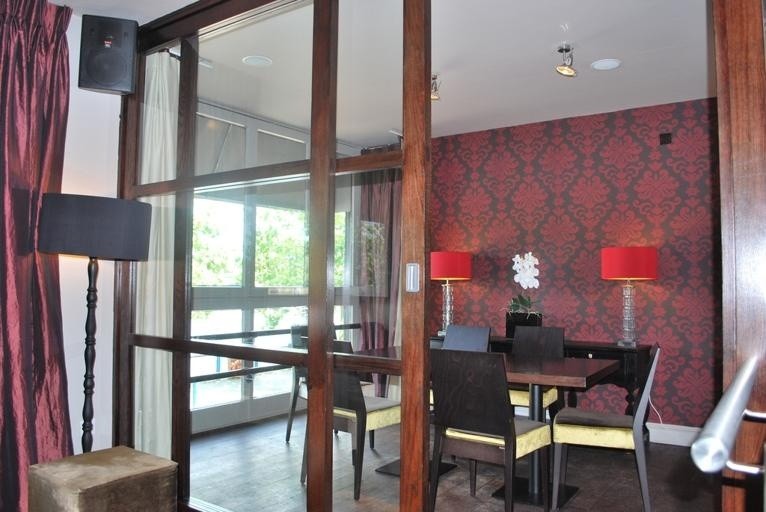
[555,44,577,77]
[38,193,152,452]
[431,74,440,99]
[601,246,658,347]
[431,252,473,336]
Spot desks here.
[354,339,621,512]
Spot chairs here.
[508,326,565,423]
[551,343,661,512]
[428,347,552,511]
[285,324,377,448]
[300,337,401,500]
[429,325,492,405]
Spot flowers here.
[505,251,541,313]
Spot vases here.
[506,311,542,337]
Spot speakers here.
[78,13,137,94]
[38,191,153,262]
